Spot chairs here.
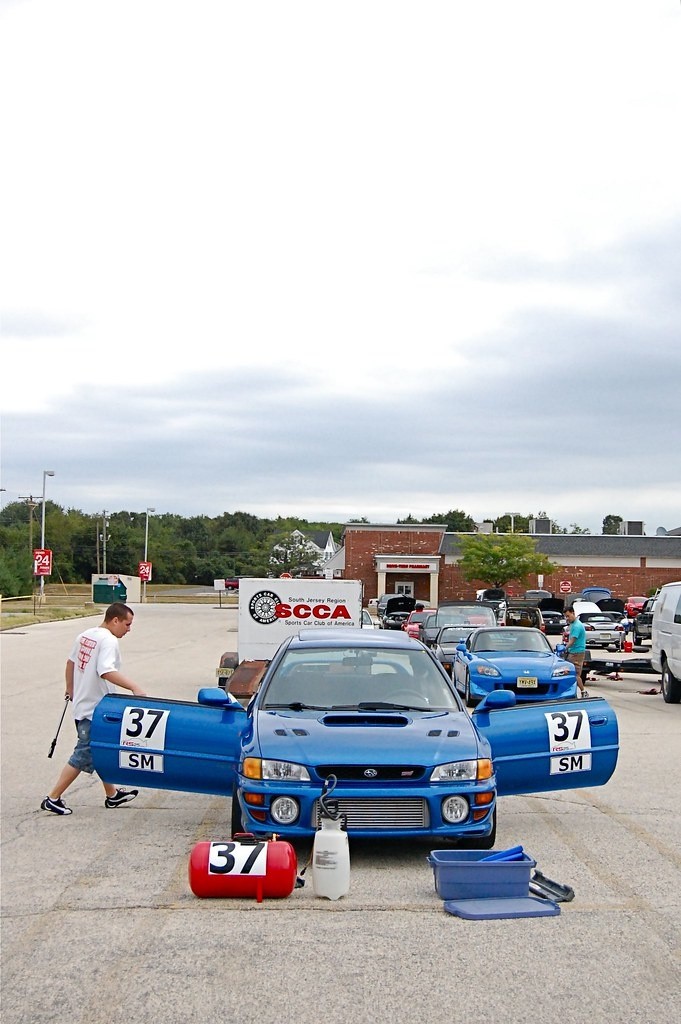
[474,634,492,650]
[514,634,536,650]
[274,669,324,704]
[363,672,406,701]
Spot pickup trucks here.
[225,578,239,590]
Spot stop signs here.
[561,582,571,592]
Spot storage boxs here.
[426,850,537,900]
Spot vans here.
[650,580,681,704]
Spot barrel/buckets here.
[624,641,632,652]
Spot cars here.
[452,625,579,705]
[89,631,619,849]
[418,614,436,645]
[377,594,416,630]
[475,586,625,634]
[573,602,625,653]
[625,596,651,617]
[431,624,484,670]
[362,609,376,629]
[404,609,437,638]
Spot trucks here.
[436,602,505,631]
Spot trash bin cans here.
[93,578,127,604]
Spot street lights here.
[99,534,112,574]
[39,470,55,600]
[142,507,156,600]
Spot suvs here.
[632,598,657,645]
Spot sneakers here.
[581,691,589,698]
[105,787,138,807]
[41,796,73,815]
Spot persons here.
[562,607,589,698]
[41,602,146,815]
